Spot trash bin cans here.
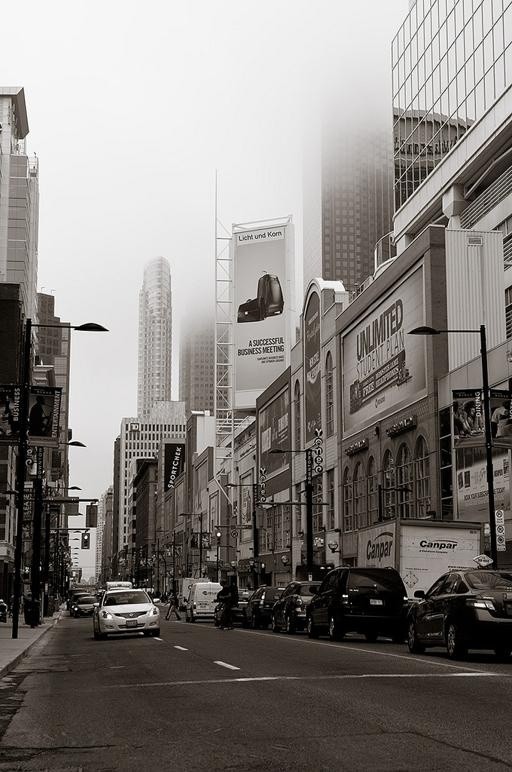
[24,600,42,628]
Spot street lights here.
[10,320,112,643]
[112,510,205,600]
[265,447,316,583]
[403,323,499,570]
[223,482,261,588]
[25,434,87,628]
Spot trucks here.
[354,518,487,606]
[181,581,221,624]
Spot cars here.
[89,587,161,640]
[64,578,154,619]
[232,560,512,664]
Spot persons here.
[218,586,239,630]
[216,584,230,608]
[166,592,182,621]
